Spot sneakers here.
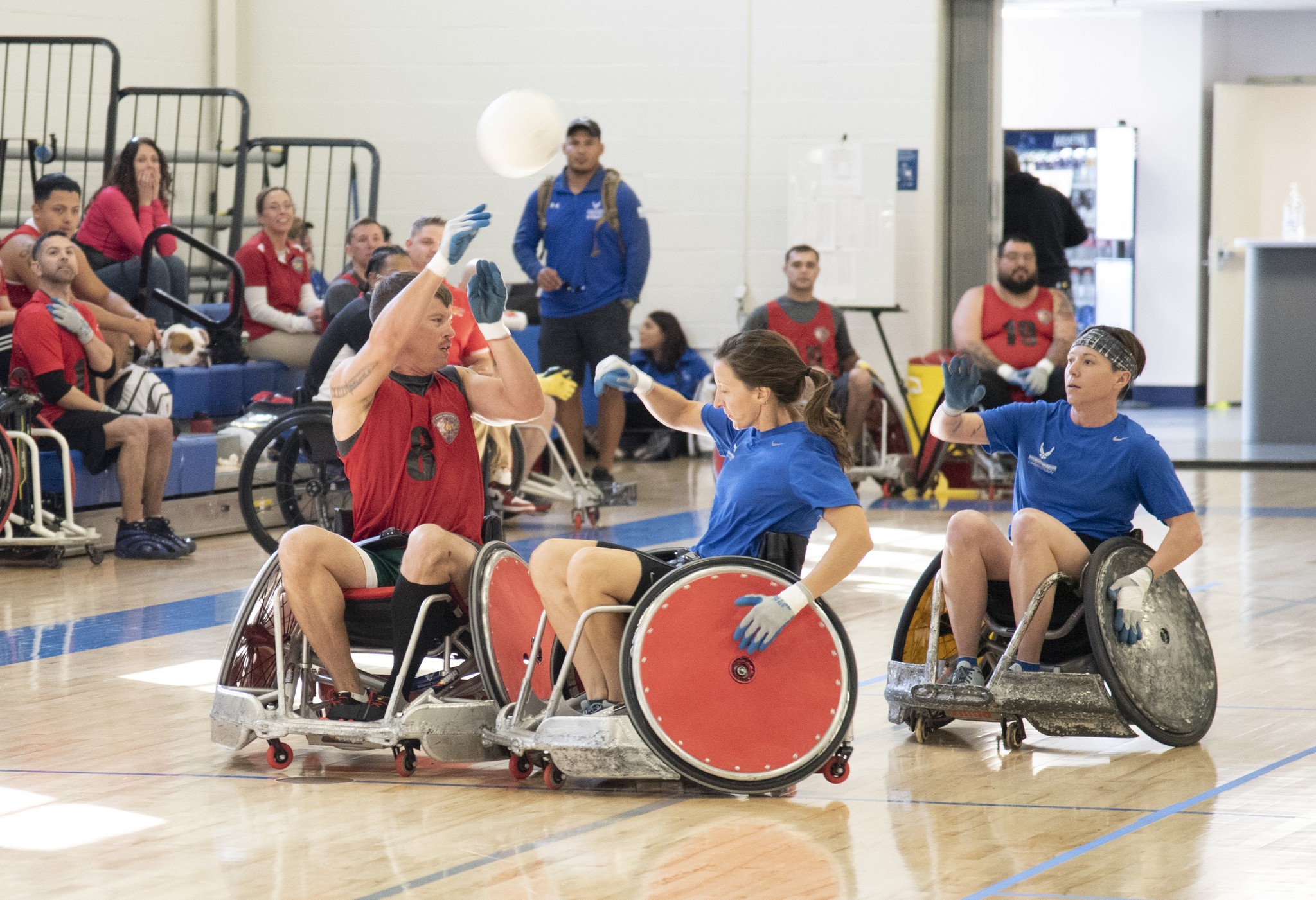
[146,517,196,555]
[115,517,188,560]
[487,480,535,513]
[318,688,375,722]
[348,690,391,723]
[519,489,551,511]
[580,700,619,715]
[593,467,617,503]
[952,660,985,687]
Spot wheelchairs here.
[211,511,555,777]
[482,531,859,797]
[0,367,104,569]
[885,529,1218,754]
[238,385,602,555]
[711,373,1019,496]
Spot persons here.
[931,326,1204,687]
[1002,149,1089,300]
[0,118,872,722]
[951,233,1075,469]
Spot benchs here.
[1,303,304,512]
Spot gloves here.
[538,367,578,402]
[427,204,490,274]
[1108,567,1154,643]
[734,580,813,652]
[943,356,986,413]
[594,353,639,399]
[44,297,94,344]
[996,363,1026,388]
[1016,358,1056,396]
[467,258,511,341]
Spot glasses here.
[999,252,1036,262]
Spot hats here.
[567,118,601,138]
